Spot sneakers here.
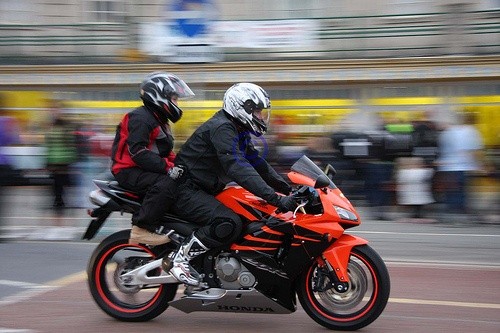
[129,225,171,246]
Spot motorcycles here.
[80,155,391,330]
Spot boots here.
[162,231,210,287]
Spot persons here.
[112,71,194,246]
[45,119,78,210]
[163,83,297,287]
[362,107,484,221]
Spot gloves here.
[167,165,185,182]
[266,194,297,214]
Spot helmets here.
[223,83,271,137]
[140,72,195,123]
